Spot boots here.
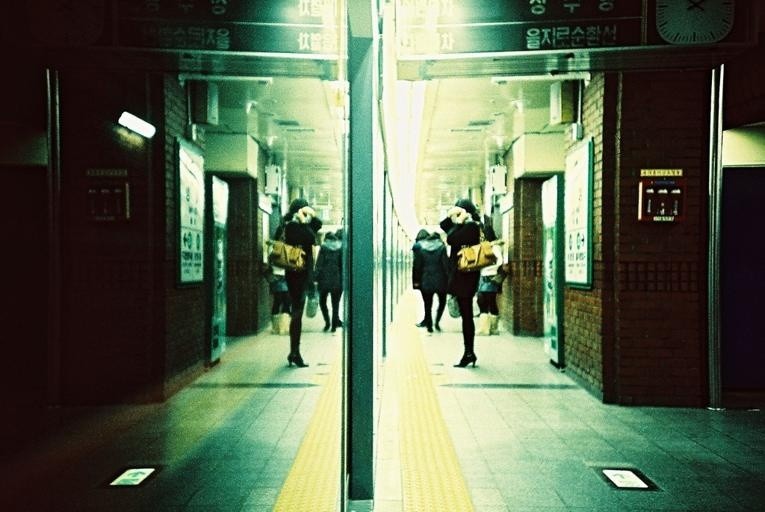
[269,313,291,335]
[477,310,502,337]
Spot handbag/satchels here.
[307,294,318,318]
[447,297,463,319]
[456,240,496,271]
[265,239,305,272]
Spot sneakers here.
[322,316,344,333]
[416,320,444,336]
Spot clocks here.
[655,0,736,46]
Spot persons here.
[412,227,429,328]
[315,231,342,334]
[475,221,502,337]
[413,231,449,332]
[332,228,345,327]
[438,198,482,368]
[266,224,292,336]
[281,197,323,369]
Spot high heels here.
[287,350,310,369]
[453,352,476,371]
